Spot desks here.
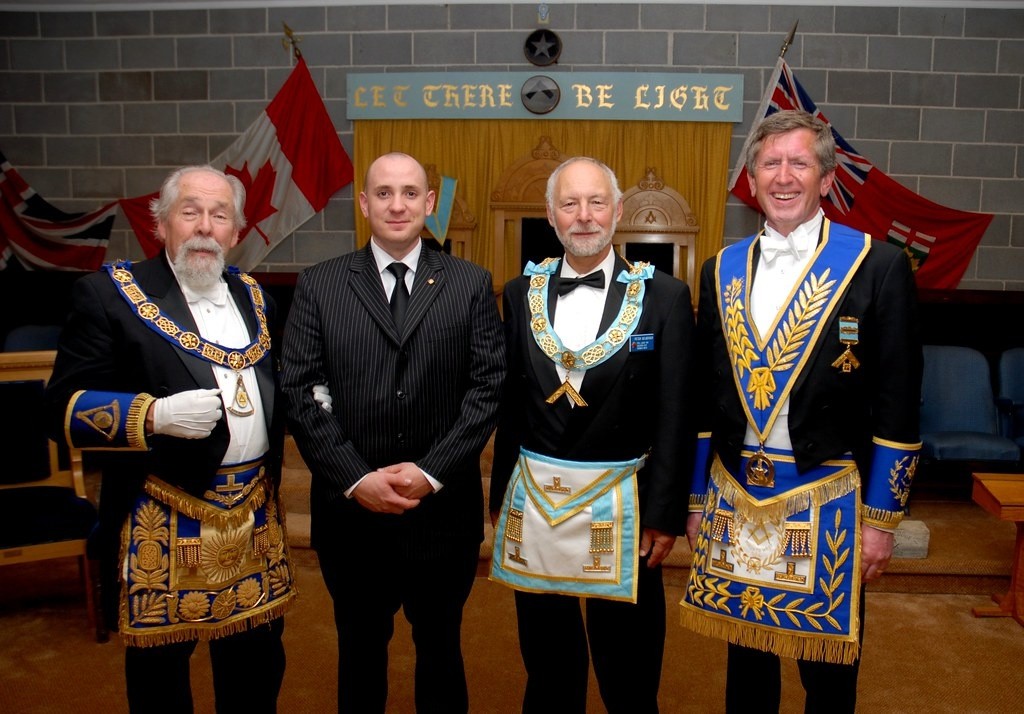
[971,473,1024,628]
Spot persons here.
[280,150,506,714]
[488,156,697,714]
[685,109,926,714]
[34,164,333,714]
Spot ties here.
[386,262,411,337]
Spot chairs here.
[0,379,111,645]
[919,345,1024,462]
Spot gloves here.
[313,381,333,413]
[153,388,223,440]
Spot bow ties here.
[180,281,228,307]
[555,269,606,297]
[759,226,808,264]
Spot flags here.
[119,59,355,275]
[0,151,119,272]
[726,56,996,301]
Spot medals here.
[831,315,862,375]
[746,448,776,488]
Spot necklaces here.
[101,259,271,416]
[523,255,656,406]
